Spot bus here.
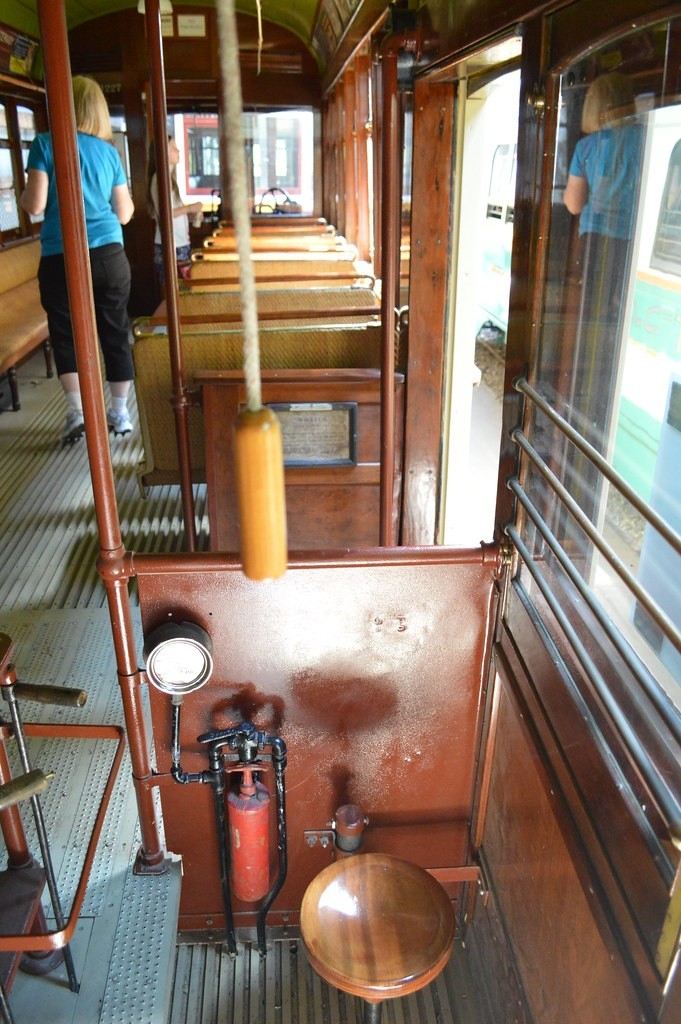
[459,116,681,494]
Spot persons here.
[563,73,643,357]
[21,76,135,448]
[146,135,203,295]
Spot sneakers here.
[107,409,133,433]
[63,409,84,442]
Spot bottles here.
[192,202,203,227]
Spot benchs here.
[0,218,402,501]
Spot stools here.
[302,853,457,1024]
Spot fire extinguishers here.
[225,763,271,902]
